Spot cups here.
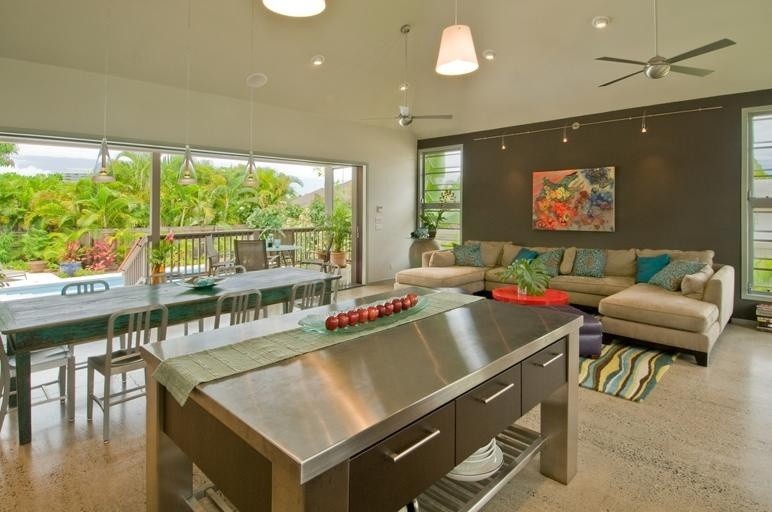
[275,239,281,249]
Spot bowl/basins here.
[464,439,496,468]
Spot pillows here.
[428,241,714,292]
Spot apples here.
[325,294,417,331]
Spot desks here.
[140,286,584,512]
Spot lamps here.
[435,0,480,76]
[262,0,326,18]
[91,0,258,188]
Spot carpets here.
[578,339,681,402]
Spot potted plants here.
[409,189,455,269]
[310,206,352,266]
[494,258,551,296]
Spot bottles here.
[268,234,273,250]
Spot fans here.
[595,0,736,88]
[360,24,453,127]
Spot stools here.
[545,304,604,359]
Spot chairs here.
[0,234,343,444]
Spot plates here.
[173,274,230,288]
[443,444,505,482]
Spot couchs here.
[393,239,735,367]
